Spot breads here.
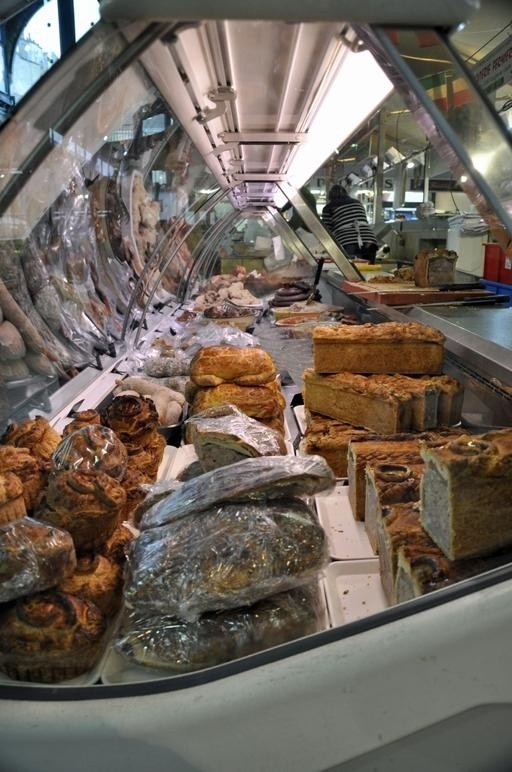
[2,320,512,693]
[2,242,138,381]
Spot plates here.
[275,316,306,326]
[356,265,381,271]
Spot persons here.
[322,185,378,265]
[276,185,318,234]
